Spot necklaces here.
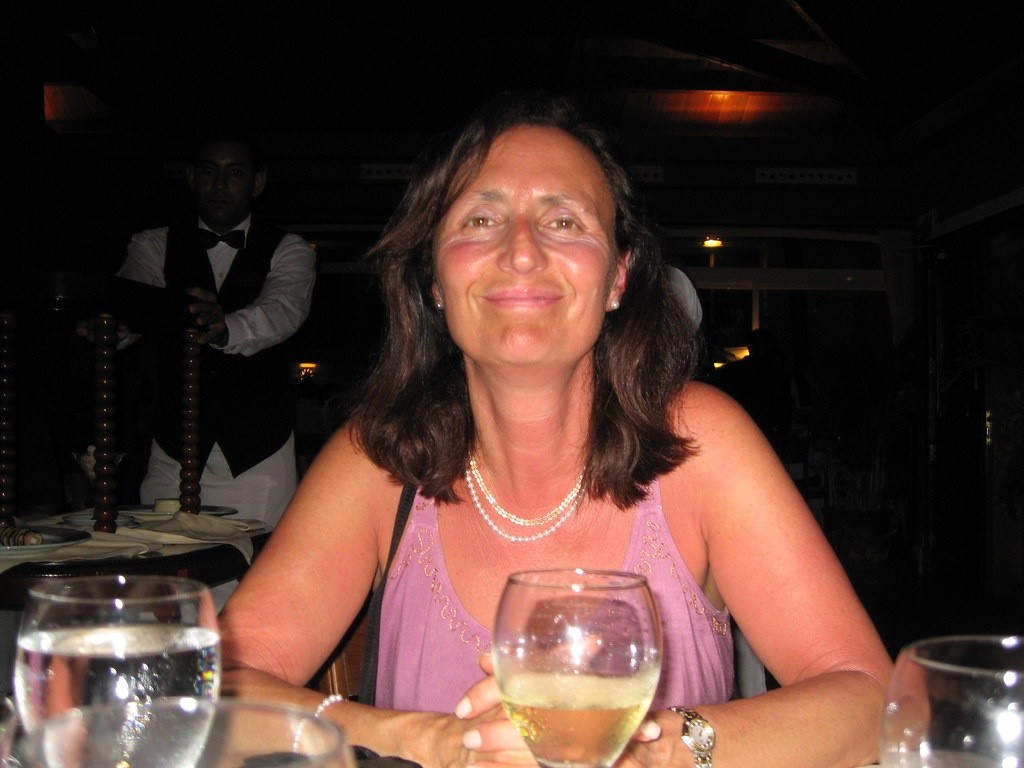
[466,445,594,542]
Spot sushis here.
[0,526,43,546]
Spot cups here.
[491,570,662,768]
[880,633,1024,768]
[12,573,222,768]
[200,696,359,768]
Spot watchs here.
[667,705,716,768]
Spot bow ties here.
[199,228,245,249]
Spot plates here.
[0,528,91,558]
[62,514,136,527]
[119,510,174,521]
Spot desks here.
[0,503,274,612]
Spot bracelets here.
[292,695,343,754]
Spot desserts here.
[154,499,181,514]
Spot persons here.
[76,126,316,704]
[212,103,929,768]
